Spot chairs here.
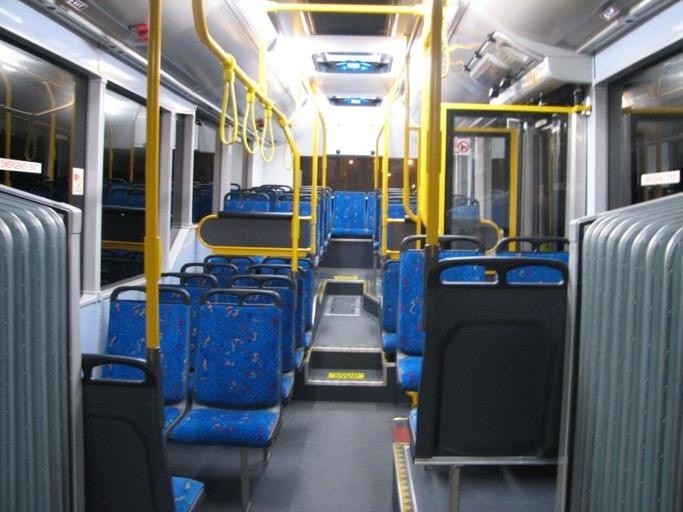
[367,187,417,269]
[380,260,400,363]
[327,191,374,242]
[224,184,293,213]
[396,234,486,409]
[408,256,569,511]
[274,186,332,274]
[99,286,191,438]
[446,194,480,219]
[495,236,570,284]
[81,352,206,512]
[159,254,314,405]
[165,288,283,512]
